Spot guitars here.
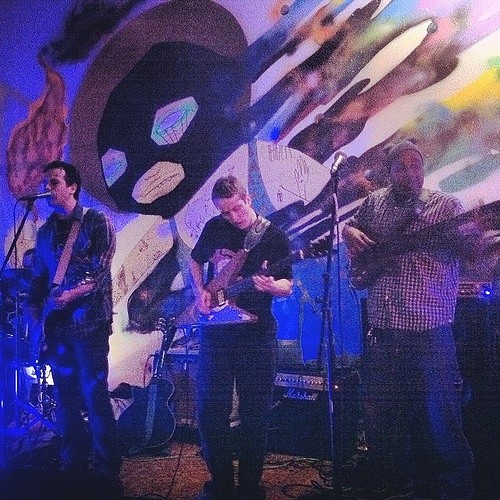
[116,316,178,449]
[21,269,97,359]
[347,200,500,291]
[173,233,339,329]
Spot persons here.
[190,175,294,500]
[22,248,34,267]
[28,159,117,500]
[342,141,486,500]
[330,151,376,207]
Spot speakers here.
[270,387,353,459]
[151,350,240,429]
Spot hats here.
[384,137,424,174]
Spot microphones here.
[330,152,347,176]
[19,191,56,203]
